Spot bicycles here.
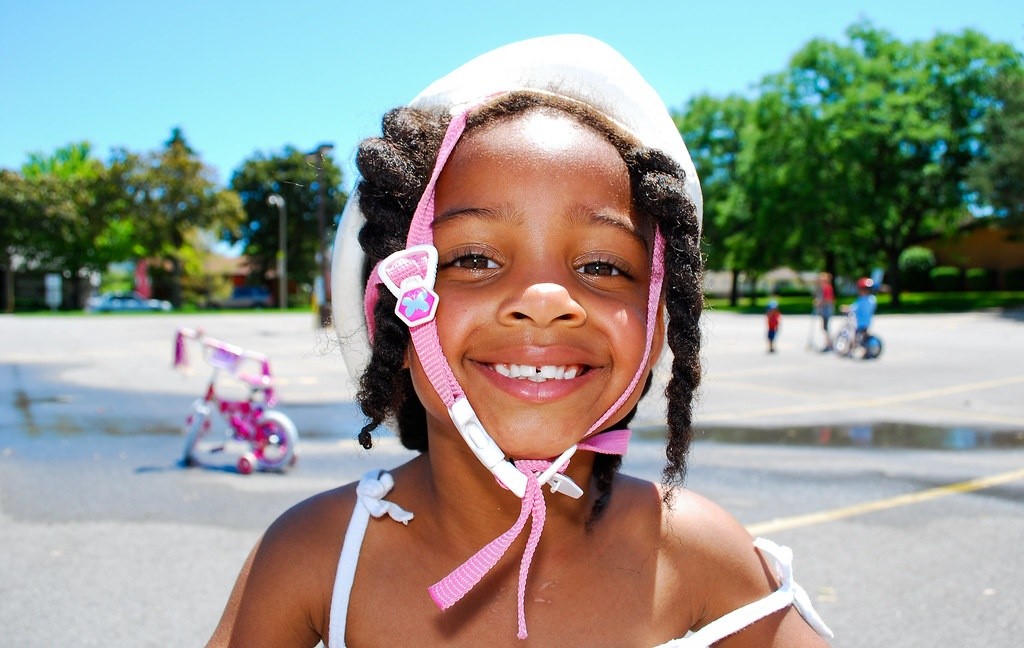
[173,325,300,475]
[831,304,882,360]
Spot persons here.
[813,271,834,352]
[837,278,877,358]
[765,300,782,352]
[205,35,834,647]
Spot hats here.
[330,34,704,438]
[857,277,872,289]
[766,301,778,312]
